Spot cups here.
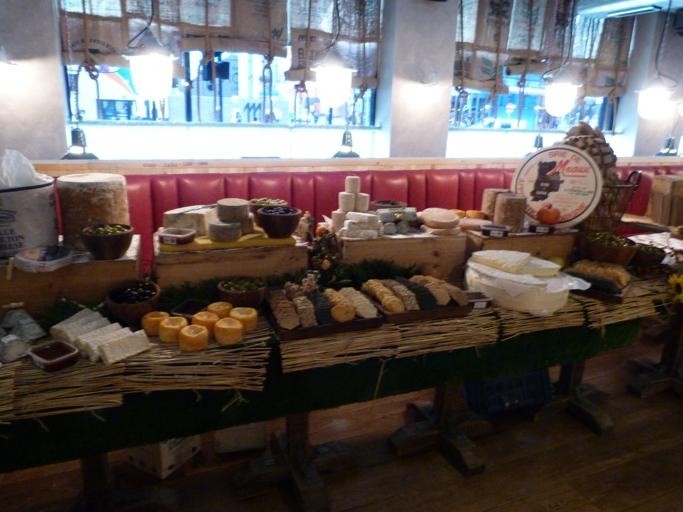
[495,194,526,234]
[483,187,509,219]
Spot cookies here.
[267,275,470,330]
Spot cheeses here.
[464,249,568,315]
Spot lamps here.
[534,61,585,118]
[116,29,186,103]
[310,47,359,110]
[632,72,677,122]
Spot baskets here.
[575,171,641,230]
[467,368,551,419]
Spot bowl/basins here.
[257,205,301,238]
[217,279,267,308]
[79,222,133,259]
[577,229,667,270]
[104,278,160,321]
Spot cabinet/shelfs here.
[1,215,682,509]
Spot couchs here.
[52,162,682,276]
